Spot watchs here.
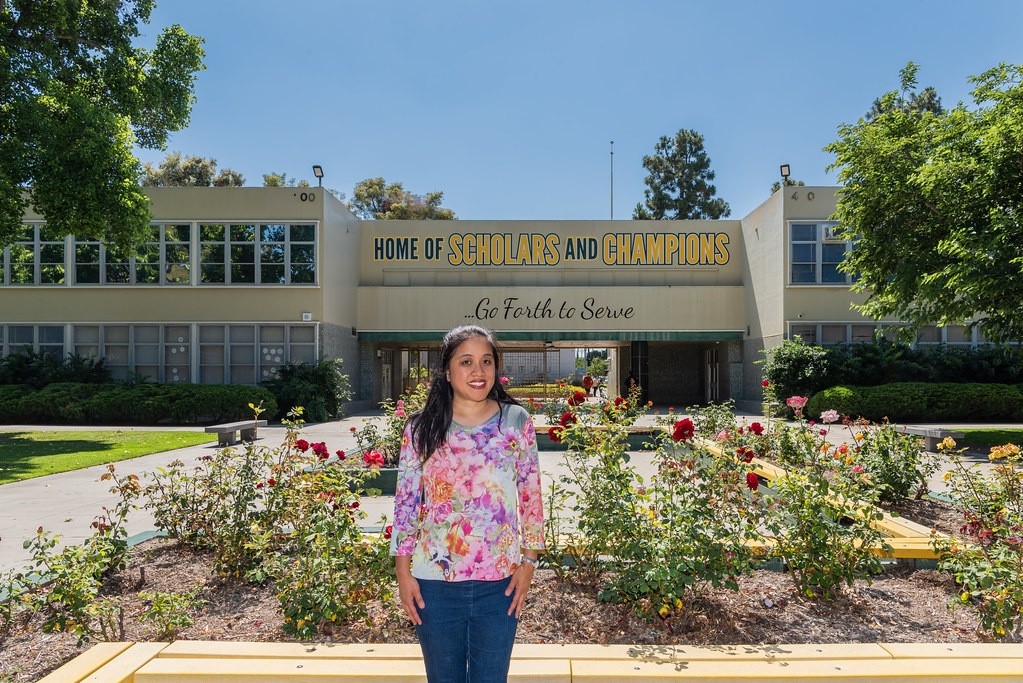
[521,556,539,570]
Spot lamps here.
[780,164,790,182]
[312,165,324,186]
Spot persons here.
[389,325,543,683]
[592,376,598,397]
[583,372,594,401]
[624,371,639,388]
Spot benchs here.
[205,420,267,446]
[892,424,965,451]
